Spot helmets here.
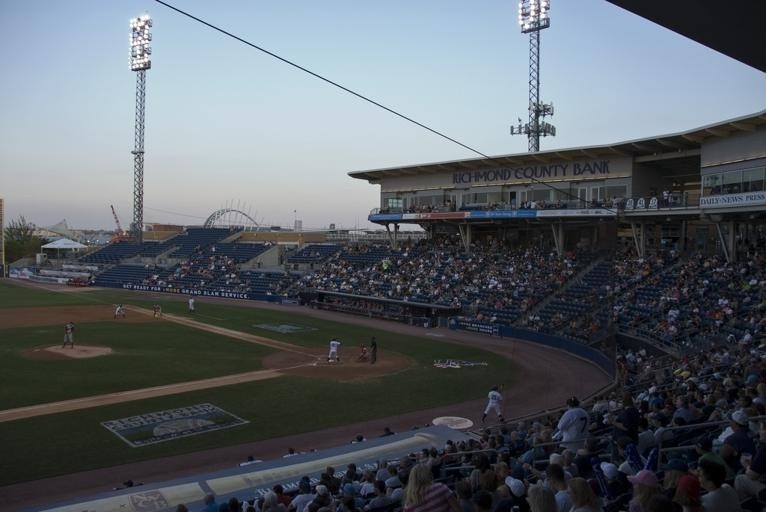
[491,384,499,390]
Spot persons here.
[62,320,75,349]
[114,303,126,319]
[141,191,765,511]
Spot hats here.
[315,395,746,511]
